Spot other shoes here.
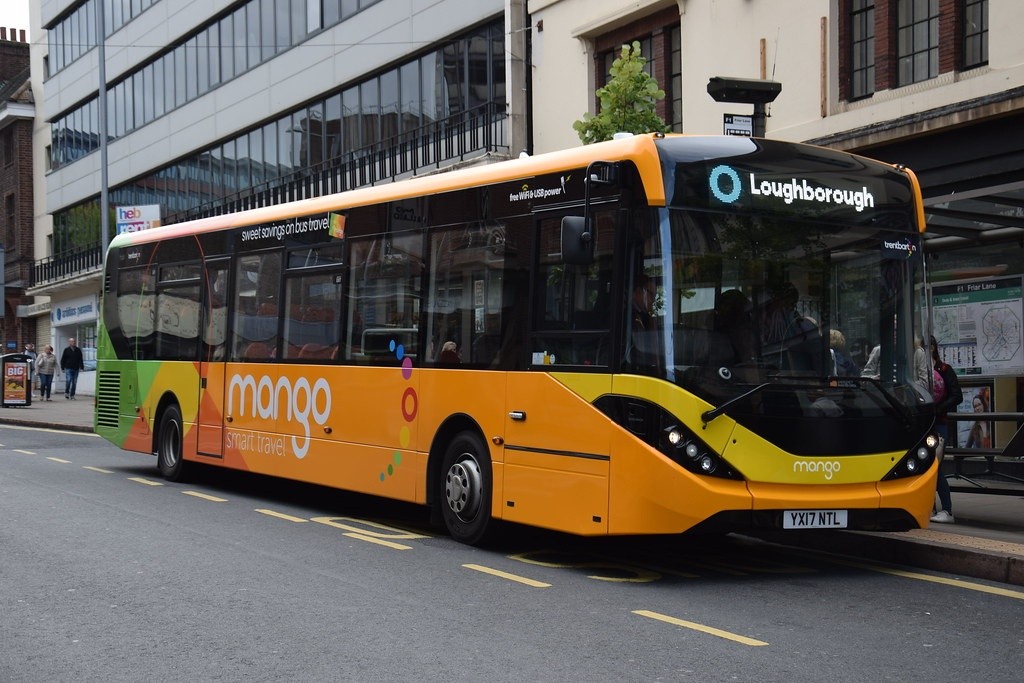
[71,395,75,400]
[40,396,44,401]
[64,392,69,399]
[46,397,52,401]
[31,393,36,397]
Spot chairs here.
[271,344,299,359]
[244,343,271,359]
[298,343,325,360]
[256,302,335,322]
[330,344,339,360]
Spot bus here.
[92,130,940,548]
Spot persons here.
[439,273,962,522]
[20,344,38,398]
[967,397,992,450]
[34,345,60,401]
[60,337,84,400]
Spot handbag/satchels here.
[38,357,44,367]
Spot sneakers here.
[930,509,955,523]
